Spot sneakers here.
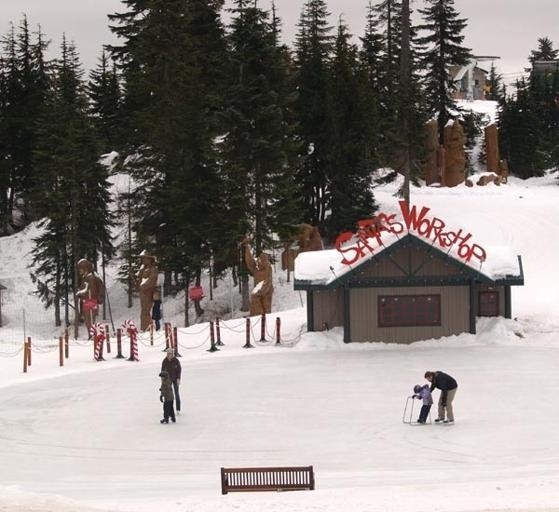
[161,418,168,423]
[443,418,454,423]
[171,418,175,422]
[435,418,444,422]
[418,419,425,423]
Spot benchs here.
[221,465,315,495]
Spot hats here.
[414,385,421,393]
[167,348,175,353]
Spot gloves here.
[418,396,422,400]
[160,396,164,402]
[413,394,417,399]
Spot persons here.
[160,346,182,414]
[241,237,274,316]
[424,370,458,424]
[128,249,159,332]
[158,371,177,424]
[74,257,105,342]
[411,384,432,423]
[295,224,321,252]
